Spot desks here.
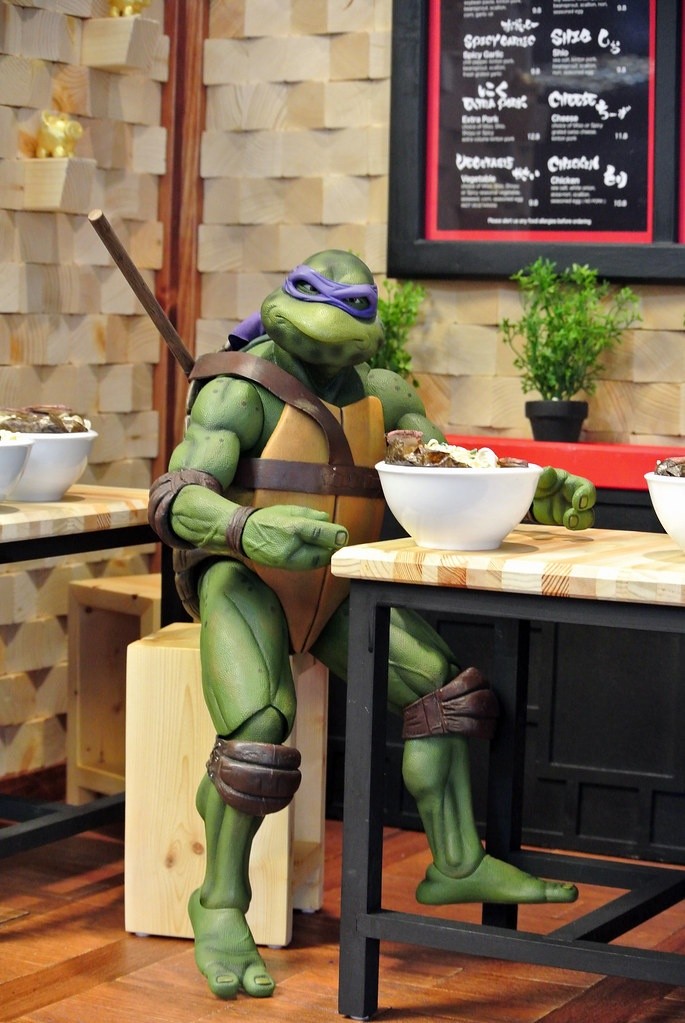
[329,524,685,1023]
[0,485,159,793]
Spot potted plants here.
[495,254,644,442]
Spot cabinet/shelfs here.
[446,435,685,533]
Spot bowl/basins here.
[644,472,685,547]
[0,437,35,502]
[6,430,98,502]
[375,460,543,551]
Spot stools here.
[66,573,332,949]
